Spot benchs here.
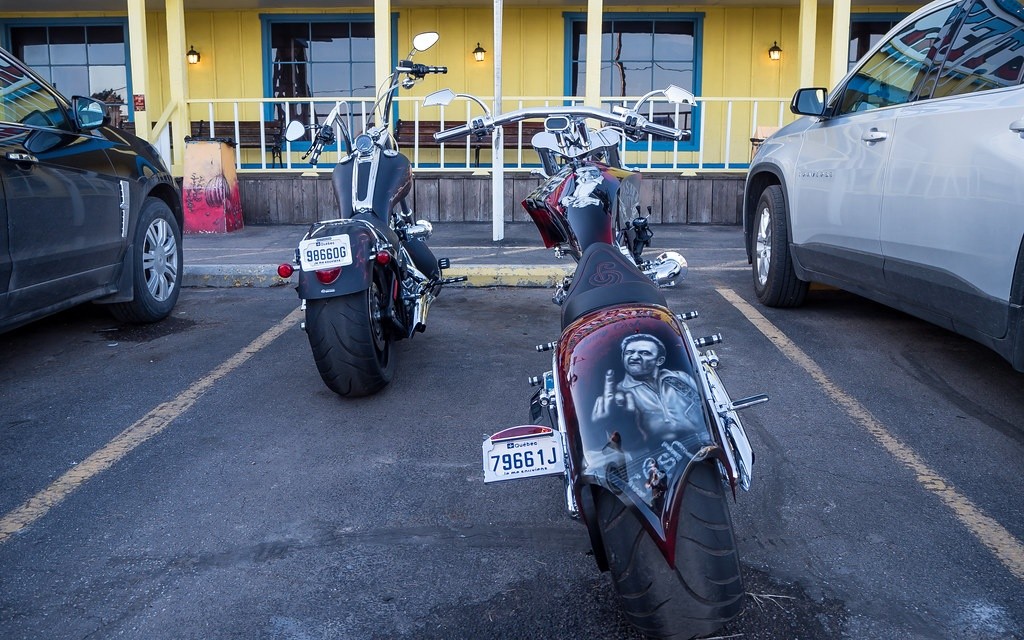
[393,118,562,167]
[120,121,286,169]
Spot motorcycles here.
[421,84,770,640]
[278,32,468,401]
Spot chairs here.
[749,124,780,164]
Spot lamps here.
[769,41,783,60]
[471,42,488,63]
[185,46,201,65]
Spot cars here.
[0,44,186,335]
[742,0,1024,376]
